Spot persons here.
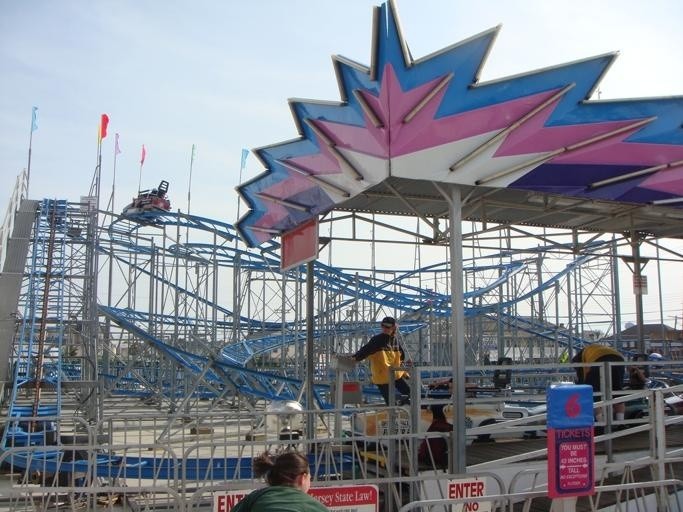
[226,450,330,511]
[628,365,647,389]
[570,343,628,430]
[347,315,411,407]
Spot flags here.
[26,103,40,205]
[95,112,108,207]
[110,133,121,219]
[236,146,249,224]
[137,144,146,194]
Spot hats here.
[382,318,395,328]
